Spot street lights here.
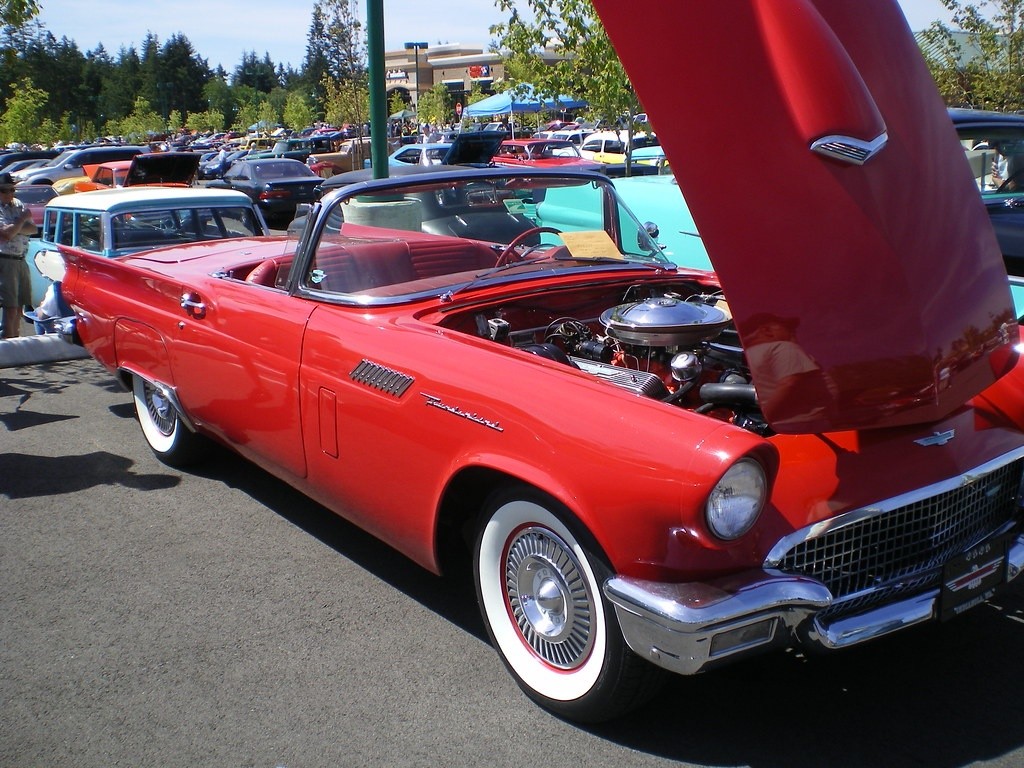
[404,42,428,134]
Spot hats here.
[0,173,17,185]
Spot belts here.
[0,253,24,260]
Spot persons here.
[0,171,38,338]
[449,116,455,131]
[33,279,61,335]
[421,123,437,144]
[992,148,1022,193]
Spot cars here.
[1,114,1024,403]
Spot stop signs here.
[456,103,462,115]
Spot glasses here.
[0,187,15,194]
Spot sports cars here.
[57,0,1024,730]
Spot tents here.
[389,109,418,138]
[459,80,590,140]
[248,120,281,131]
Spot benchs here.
[245,240,501,290]
